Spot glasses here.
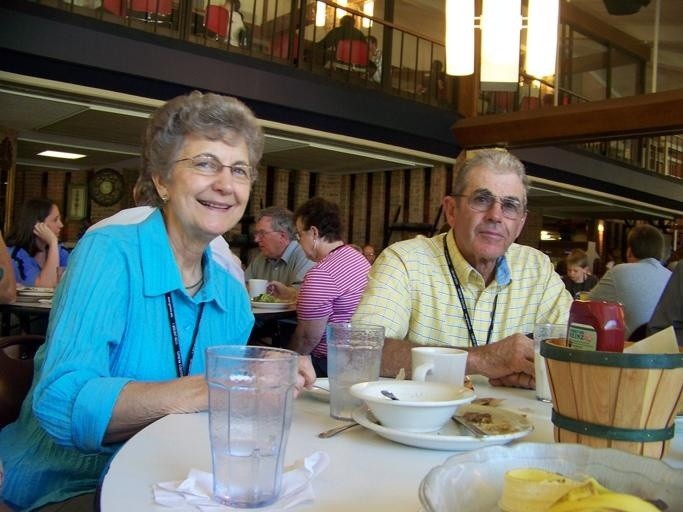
[176,153,259,184]
[254,230,282,239]
[451,193,525,220]
[294,230,303,241]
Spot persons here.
[317,15,366,65]
[216,1,247,46]
[2,196,69,302]
[555,223,681,346]
[245,197,374,376]
[351,148,574,388]
[366,34,383,82]
[1,92,316,511]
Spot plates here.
[18,292,53,298]
[251,300,295,308]
[16,287,55,293]
[352,403,534,448]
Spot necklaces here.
[186,278,202,289]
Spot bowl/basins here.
[416,443,682,512]
[349,379,477,433]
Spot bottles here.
[566,300,625,352]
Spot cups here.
[205,345,298,509]
[534,323,568,404]
[248,279,274,296]
[409,346,468,386]
[327,322,385,421]
